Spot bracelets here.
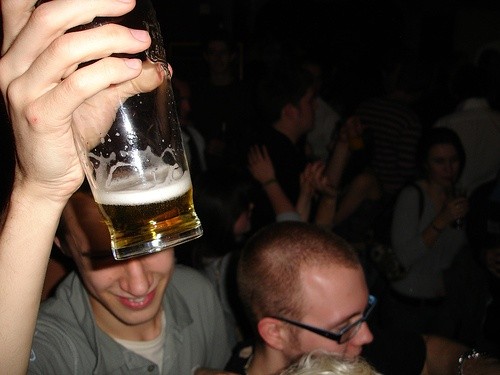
[261,178,278,187]
[432,219,445,232]
[457,348,491,375]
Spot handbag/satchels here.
[368,233,408,282]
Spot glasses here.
[263,296,376,345]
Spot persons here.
[224,220,500,375]
[0,0,243,375]
[171,36,500,358]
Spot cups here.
[445,191,468,231]
[34,1,204,260]
